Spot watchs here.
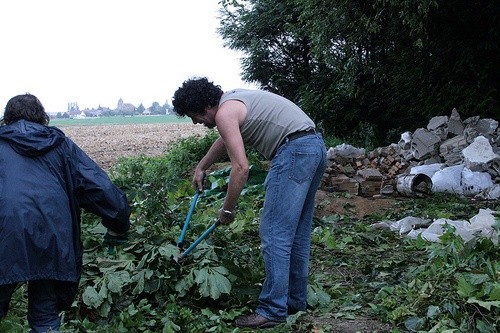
[222,208,235,216]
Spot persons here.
[0,92,134,333]
[171,76,328,329]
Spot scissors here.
[175,173,228,263]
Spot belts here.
[278,129,319,147]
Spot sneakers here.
[235,311,277,329]
[287,303,299,315]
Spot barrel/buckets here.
[395,174,432,198]
